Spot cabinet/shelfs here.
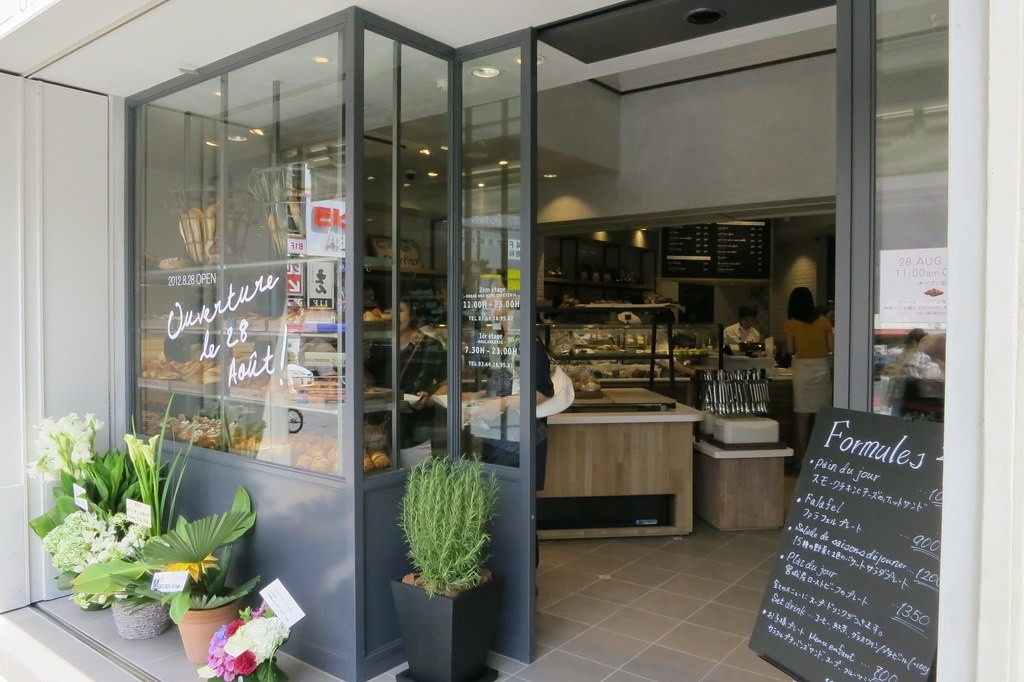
[137,252,794,540]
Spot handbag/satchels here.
[511,339,575,417]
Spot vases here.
[111,600,172,640]
[176,586,244,663]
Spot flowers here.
[196,601,292,682]
[25,391,260,624]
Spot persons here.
[817,306,836,369]
[368,292,447,471]
[880,329,932,418]
[785,287,835,473]
[722,304,760,348]
[460,272,554,603]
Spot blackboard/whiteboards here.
[746,403,946,682]
[654,221,773,283]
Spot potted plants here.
[389,452,506,682]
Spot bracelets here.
[503,396,510,409]
